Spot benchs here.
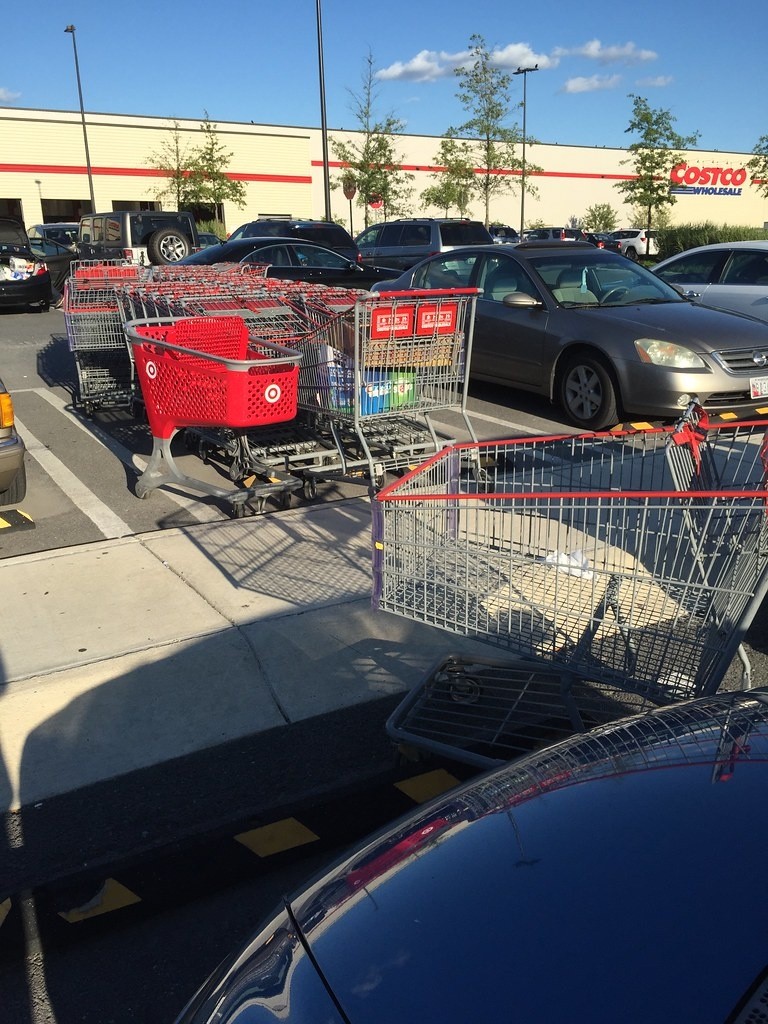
[433,269,490,295]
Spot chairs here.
[553,269,598,303]
[489,272,524,304]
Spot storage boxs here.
[309,314,450,419]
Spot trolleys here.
[372,400,768,772]
[63,256,484,519]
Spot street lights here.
[511,63,540,242]
[65,23,97,218]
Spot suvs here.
[354,217,496,272]
[607,228,666,265]
[524,226,589,242]
[0,220,79,313]
[222,220,364,264]
[78,210,201,266]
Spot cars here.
[369,238,768,431]
[517,230,533,242]
[197,231,225,253]
[483,224,522,244]
[601,239,768,322]
[26,223,79,250]
[177,685,768,1024]
[168,236,406,292]
[584,232,611,250]
